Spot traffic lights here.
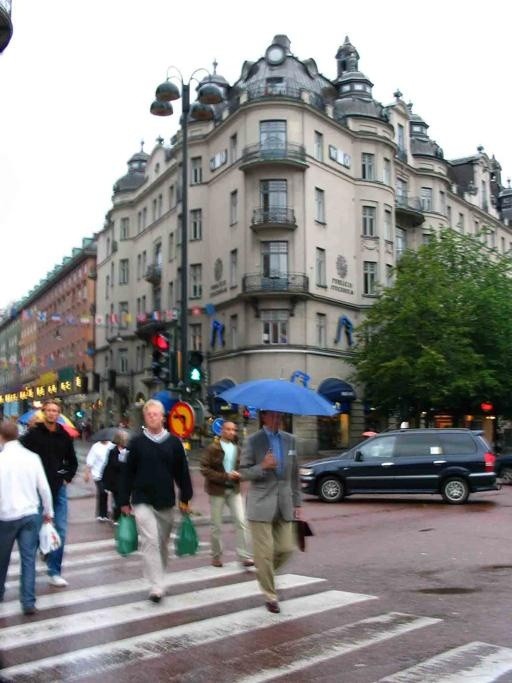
[187,350,204,384]
[153,328,173,383]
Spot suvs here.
[298,427,500,505]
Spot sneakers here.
[149,586,163,603]
[48,574,67,586]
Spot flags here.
[19,301,217,325]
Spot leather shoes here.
[24,605,39,614]
[241,557,255,566]
[212,560,222,567]
[266,601,280,612]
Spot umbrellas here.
[86,427,140,442]
[212,376,342,452]
[15,406,80,437]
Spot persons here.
[101,429,131,526]
[19,399,79,588]
[116,397,194,604]
[238,407,304,614]
[0,416,56,616]
[199,419,256,565]
[81,439,117,522]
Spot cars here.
[495,449,512,485]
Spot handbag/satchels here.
[292,519,312,551]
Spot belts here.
[224,484,234,489]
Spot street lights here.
[150,60,228,402]
